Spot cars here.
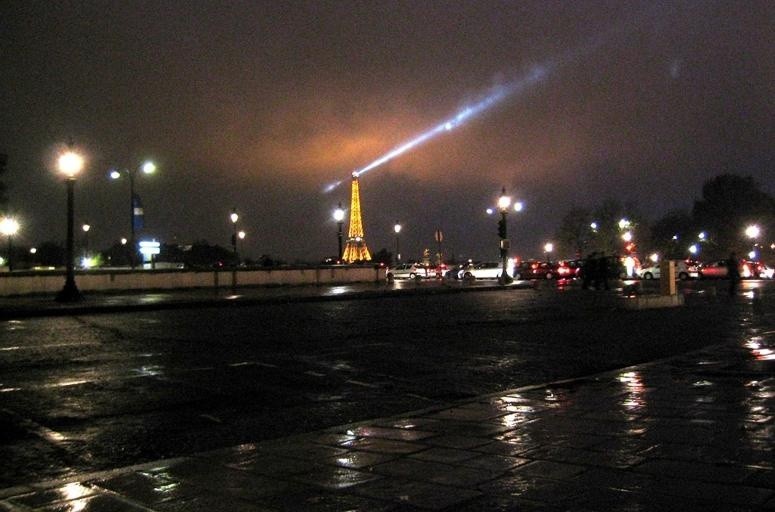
[513,259,586,281]
[386,261,503,280]
[640,259,768,281]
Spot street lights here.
[395,219,401,262]
[335,199,344,264]
[486,185,522,285]
[230,207,246,294]
[55,139,86,303]
[84,161,156,271]
[5,213,16,271]
